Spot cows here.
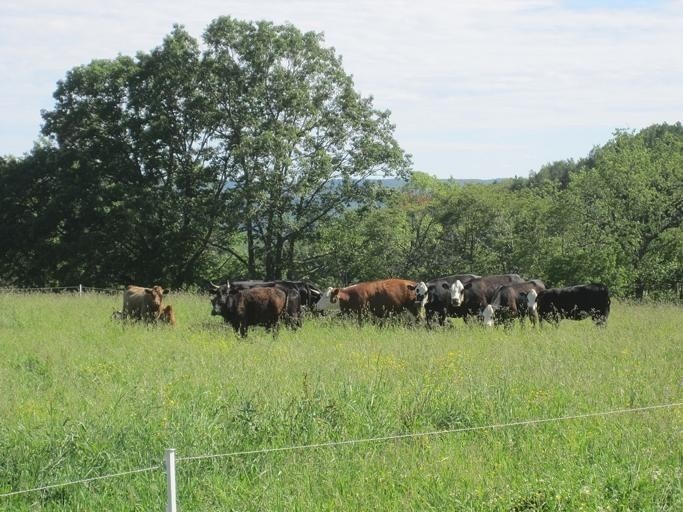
[324,279,416,326]
[520,283,611,329]
[480,280,545,327]
[407,273,481,331]
[124,285,169,321]
[443,274,528,321]
[158,305,176,324]
[210,279,323,339]
